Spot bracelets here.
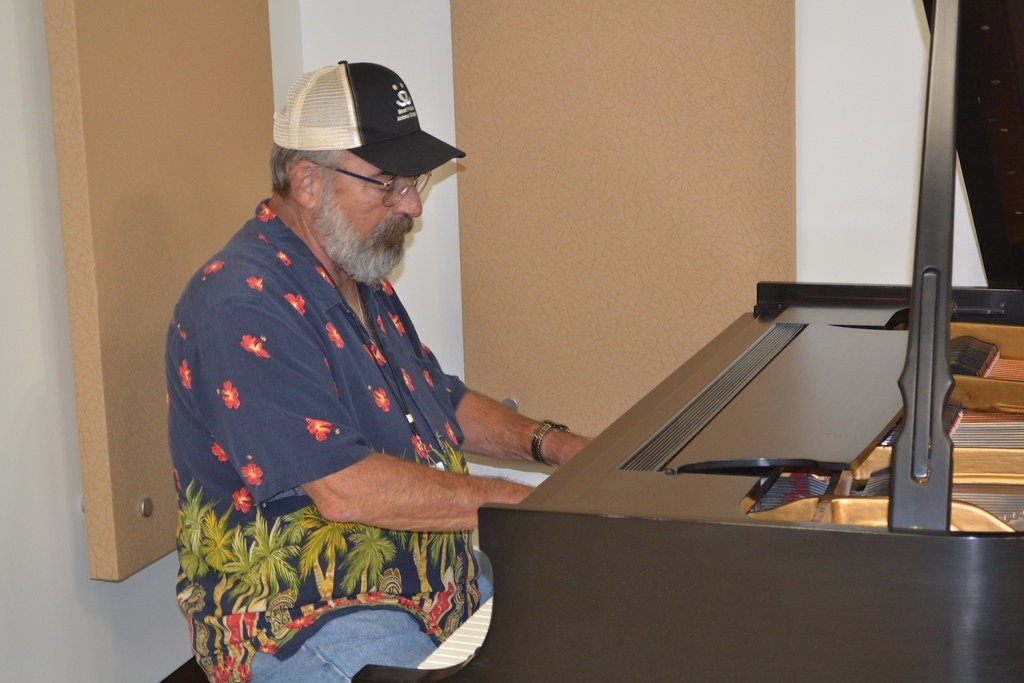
[531,419,569,467]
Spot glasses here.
[311,159,432,207]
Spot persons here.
[164,60,590,683]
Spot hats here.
[273,60,466,176]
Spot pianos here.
[353,0,1023,682]
